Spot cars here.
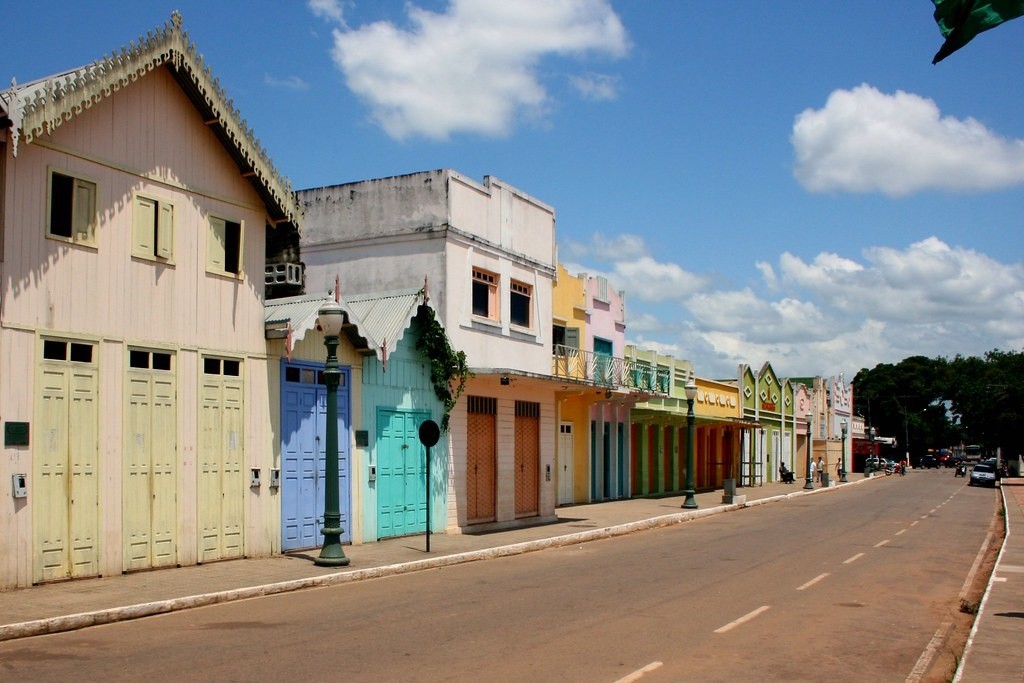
[987,458,997,464]
[969,465,996,488]
[865,458,889,469]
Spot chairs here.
[779,469,795,484]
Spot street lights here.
[803,409,815,489]
[314,289,350,568]
[840,419,848,482]
[682,371,699,509]
[870,427,876,475]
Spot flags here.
[931,0,1023,65]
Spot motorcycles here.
[954,463,967,477]
[885,465,900,476]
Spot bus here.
[964,445,981,462]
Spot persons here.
[809,457,817,481]
[780,461,796,484]
[817,457,826,482]
[834,458,843,482]
[899,459,906,477]
[956,458,966,467]
